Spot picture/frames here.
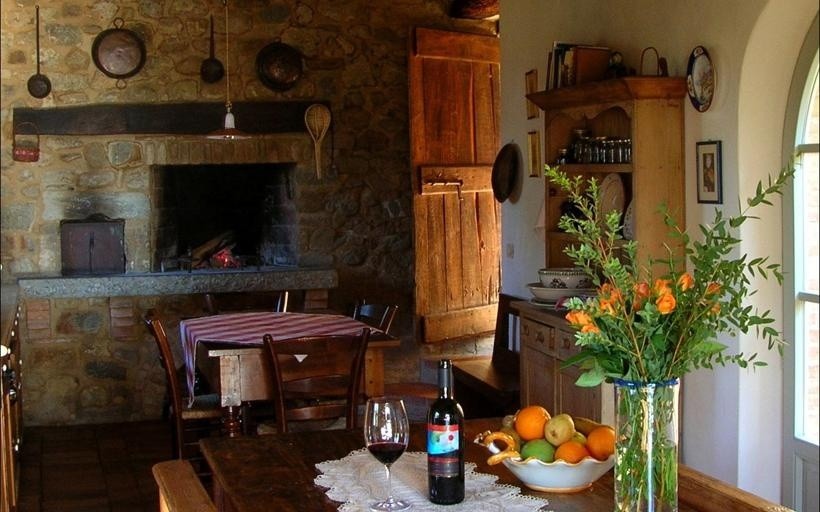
[693,137,725,206]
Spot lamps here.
[208,0,252,144]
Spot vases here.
[607,378,686,512]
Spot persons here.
[702,152,716,193]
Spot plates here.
[531,298,559,310]
[593,172,625,235]
[685,46,717,112]
[622,199,633,240]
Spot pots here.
[90,17,147,90]
[254,36,304,94]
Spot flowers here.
[534,147,817,512]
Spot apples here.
[521,438,554,462]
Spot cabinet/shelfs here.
[523,69,689,304]
[0,299,24,512]
[511,300,685,448]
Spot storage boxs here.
[56,215,128,278]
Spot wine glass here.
[365,396,412,512]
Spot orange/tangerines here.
[515,406,550,440]
[588,427,616,460]
[556,442,589,464]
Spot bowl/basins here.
[483,427,617,493]
[528,282,597,303]
[538,266,595,290]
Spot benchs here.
[566,415,794,511]
[151,453,216,512]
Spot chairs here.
[142,305,250,478]
[196,286,290,315]
[260,327,373,438]
[348,291,400,332]
[449,292,532,417]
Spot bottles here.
[425,359,466,505]
[554,128,633,164]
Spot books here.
[543,39,612,91]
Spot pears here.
[545,414,574,445]
